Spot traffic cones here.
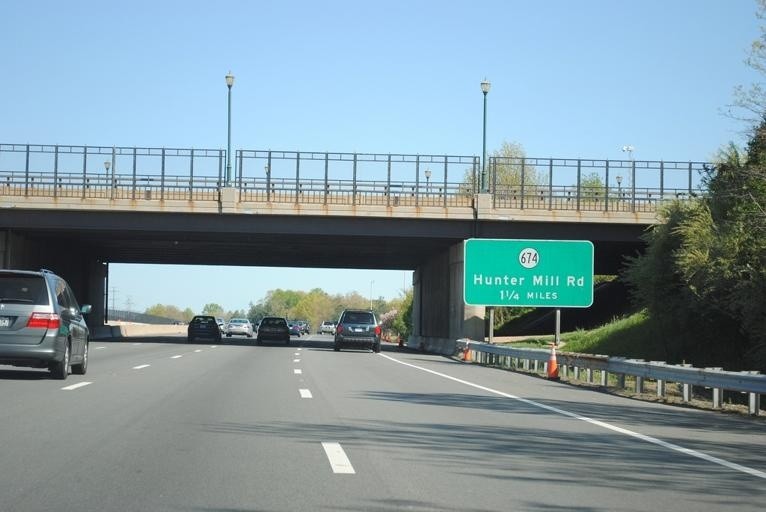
[463,335,471,363]
[546,345,559,381]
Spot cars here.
[319,308,381,353]
[187,316,310,345]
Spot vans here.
[0,268,92,380]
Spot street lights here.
[263,165,269,192]
[423,170,431,197]
[615,145,634,200]
[479,76,491,195]
[225,71,234,188]
[103,161,111,188]
